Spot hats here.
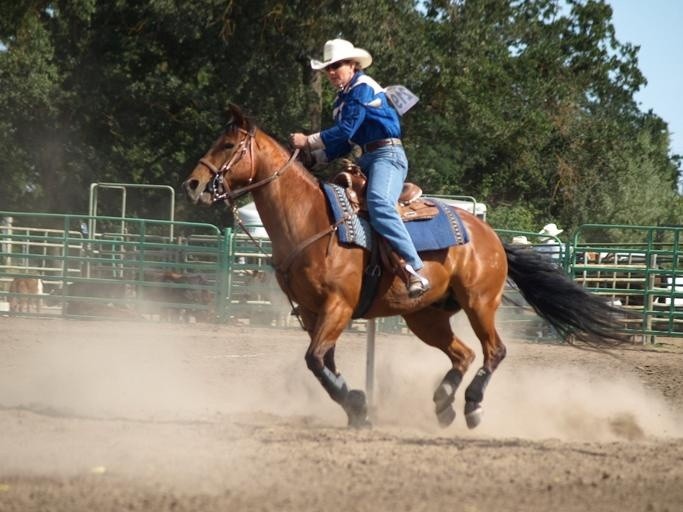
[509,223,563,245]
[309,39,372,70]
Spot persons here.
[232,255,255,319]
[161,227,207,324]
[502,236,532,333]
[264,262,292,329]
[533,223,573,339]
[289,37,430,298]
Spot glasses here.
[324,62,341,70]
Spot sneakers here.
[408,274,429,298]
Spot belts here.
[361,139,402,153]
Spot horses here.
[240,268,298,328]
[565,244,669,340]
[180,101,650,433]
[5,275,45,315]
